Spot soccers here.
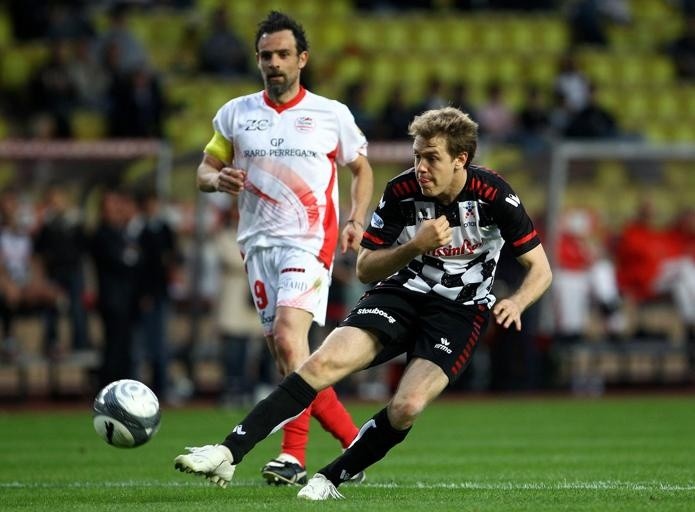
[93,379,161,450]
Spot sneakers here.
[261,454,365,501]
[174,444,236,489]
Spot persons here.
[197,8,366,488]
[172,105,554,501]
[0,1,694,409]
